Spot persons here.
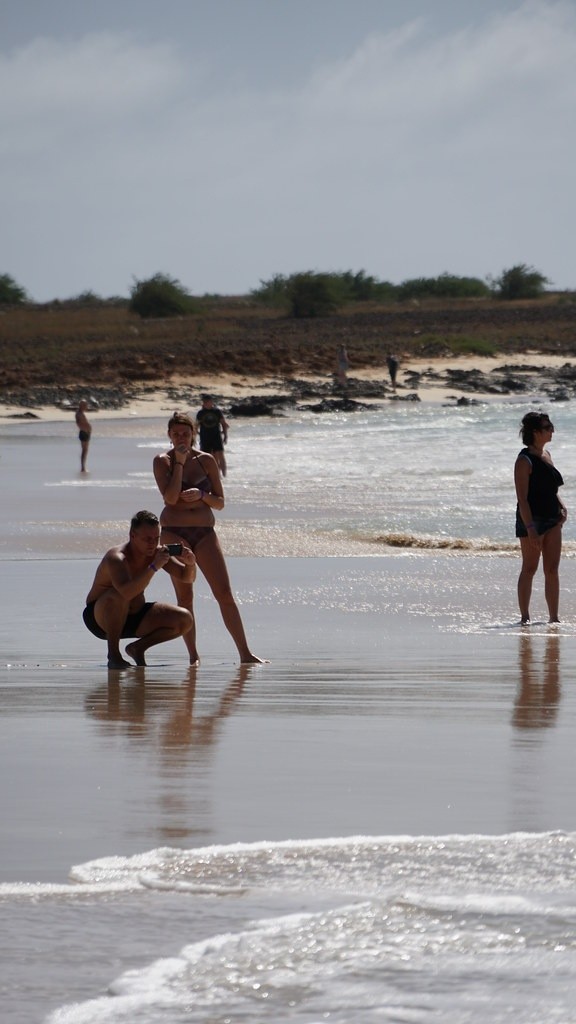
[153,410,270,668]
[75,398,92,474]
[82,509,196,669]
[386,353,400,393]
[192,394,229,478]
[336,344,350,378]
[514,410,567,625]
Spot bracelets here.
[147,562,157,572]
[199,490,204,501]
[527,523,535,529]
[174,461,185,467]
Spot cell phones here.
[165,544,182,556]
